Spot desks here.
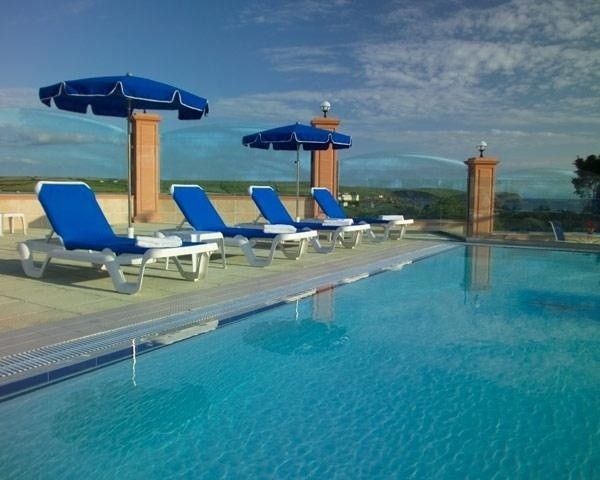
[155,227,228,274]
[562,230,599,244]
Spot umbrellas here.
[39,72,210,227]
[242,120,353,218]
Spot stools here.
[1,212,28,237]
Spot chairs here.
[547,219,576,243]
[17,180,217,294]
[309,186,413,240]
[246,184,372,254]
[166,181,319,267]
